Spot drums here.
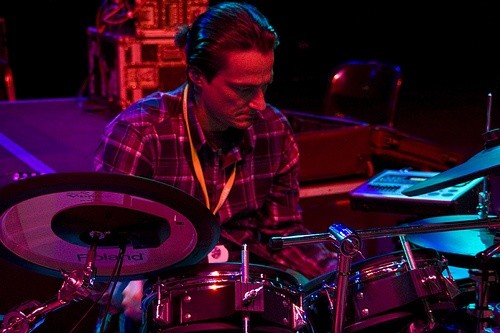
[0,292,135,333]
[140,262,300,333]
[301,247,463,333]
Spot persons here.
[90,1,338,333]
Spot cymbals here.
[0,174,221,284]
[403,215,500,258]
